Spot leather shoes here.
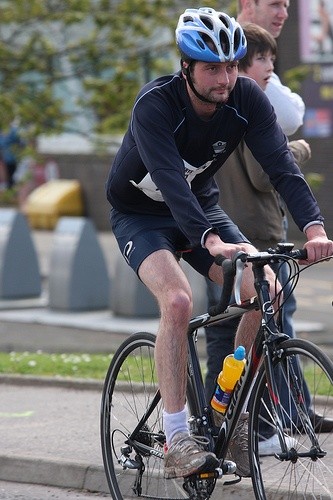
[293,408,333,433]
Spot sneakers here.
[213,409,251,479]
[163,433,220,480]
[258,434,298,457]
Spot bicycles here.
[99,241,333,500]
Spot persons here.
[200,0,333,457]
[0,121,27,189]
[108,5,333,477]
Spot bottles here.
[211,346,247,414]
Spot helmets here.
[176,8,247,63]
